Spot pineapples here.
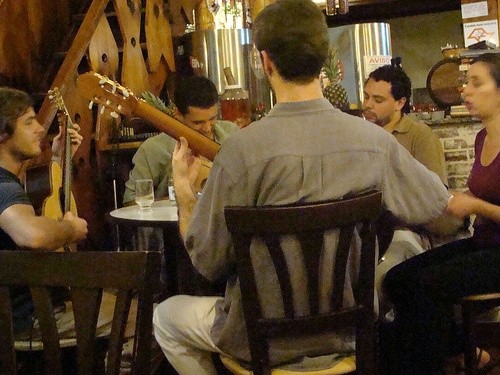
[322,50,349,112]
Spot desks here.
[107,196,192,294]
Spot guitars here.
[77,72,222,162]
[25,87,78,254]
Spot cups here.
[134,179,155,214]
[168,179,176,206]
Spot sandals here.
[460,349,494,375]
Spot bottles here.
[390,56,410,115]
[458,64,470,105]
[220,84,250,128]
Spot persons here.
[121,76,241,293]
[151,1,456,374]
[0,86,166,375]
[345,59,448,260]
[384,53,500,375]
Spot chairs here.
[0,190,500,375]
